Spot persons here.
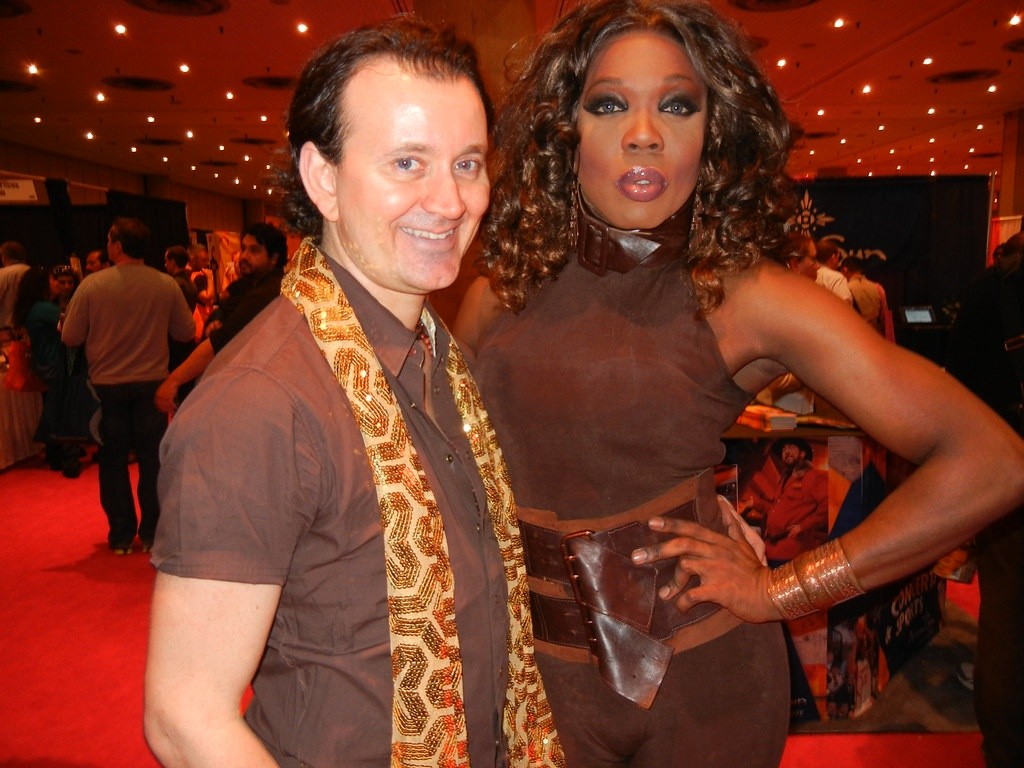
[759,229,1023,424]
[736,436,887,564]
[0,216,291,555]
[451,0,1024,768]
[143,14,530,768]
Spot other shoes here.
[111,537,154,554]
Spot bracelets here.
[767,543,868,621]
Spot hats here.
[1005,233,1023,246]
[54,266,72,274]
[771,437,812,461]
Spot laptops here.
[899,305,944,329]
